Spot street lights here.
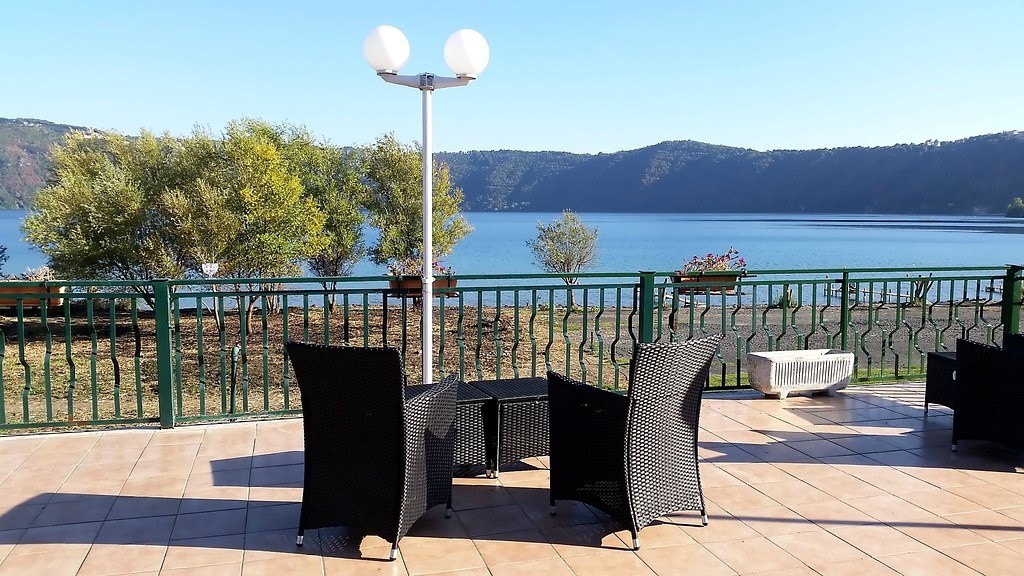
[365,25,489,385]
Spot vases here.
[746,348,855,400]
[388,280,457,296]
[0,287,66,306]
[669,277,737,292]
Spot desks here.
[469,376,550,480]
[923,351,956,418]
[404,380,493,478]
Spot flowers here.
[386,248,456,276]
[3,264,57,282]
[683,246,747,271]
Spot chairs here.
[546,330,724,551]
[952,331,1024,451]
[282,339,461,564]
